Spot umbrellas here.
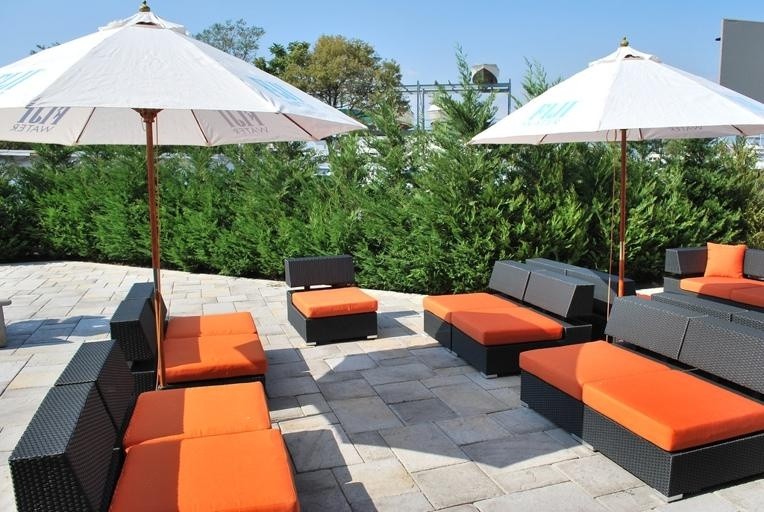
[0,3,370,390]
[463,37,764,300]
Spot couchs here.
[422,257,596,383]
[516,294,762,502]
[649,243,762,329]
[525,253,642,340]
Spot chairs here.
[54,340,272,451]
[125,283,259,339]
[9,382,300,511]
[111,297,268,402]
[285,253,379,345]
